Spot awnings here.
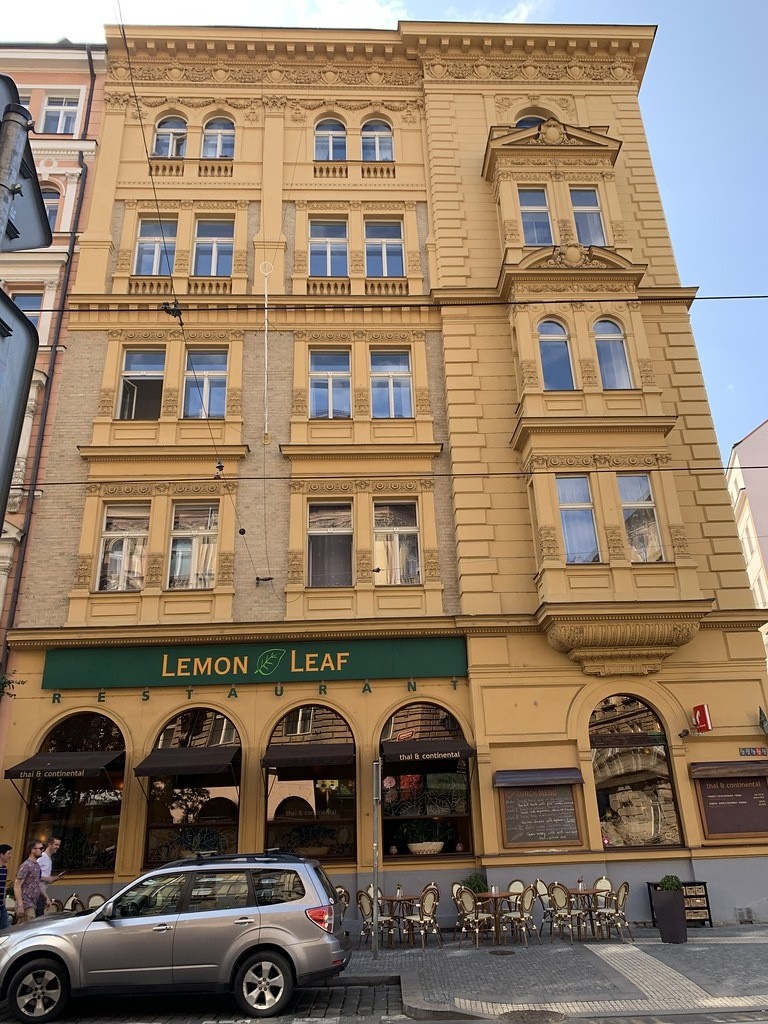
[3,742,477,807]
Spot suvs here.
[3,848,349,1023]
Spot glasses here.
[33,847,44,851]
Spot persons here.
[35,835,66,918]
[14,839,51,924]
[0,844,12,929]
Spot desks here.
[332,874,635,954]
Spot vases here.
[651,888,689,943]
[407,840,446,855]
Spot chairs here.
[4,892,107,925]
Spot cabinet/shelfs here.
[646,878,714,930]
